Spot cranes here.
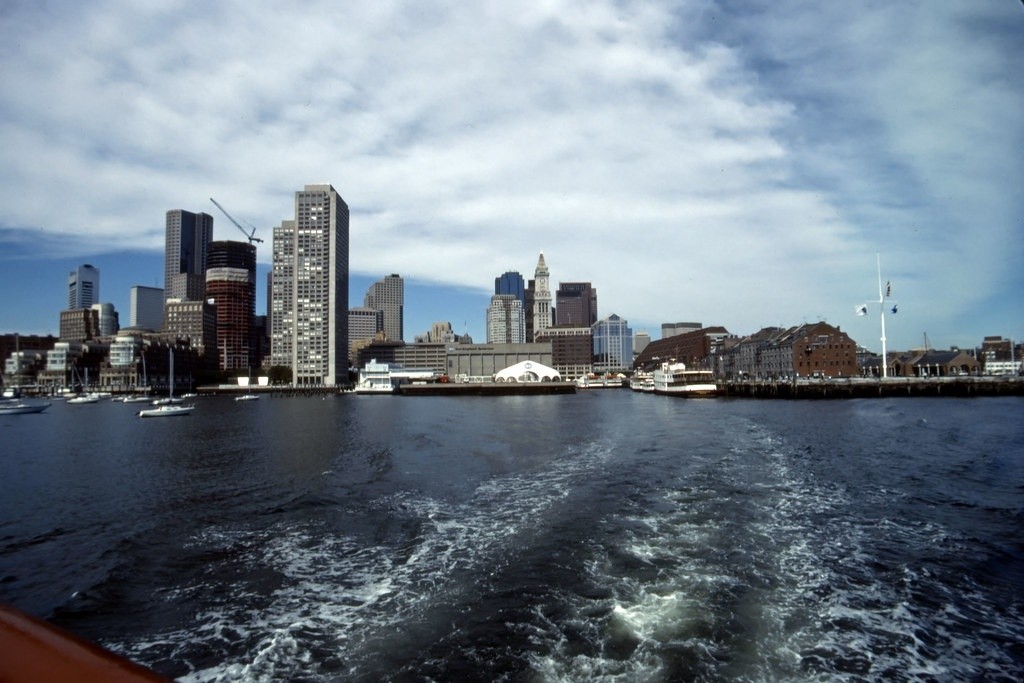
[209,196,264,245]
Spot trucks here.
[440,374,469,383]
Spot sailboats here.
[151,348,184,406]
[62,350,154,405]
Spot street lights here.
[927,364,931,376]
[862,366,866,376]
[876,365,880,377]
[935,363,940,376]
[869,366,874,378]
[855,299,899,380]
[917,364,922,377]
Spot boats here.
[138,404,195,417]
[181,393,198,398]
[630,370,655,392]
[338,389,356,394]
[653,358,719,398]
[355,358,395,394]
[0,397,52,415]
[572,373,626,388]
[234,394,260,403]
